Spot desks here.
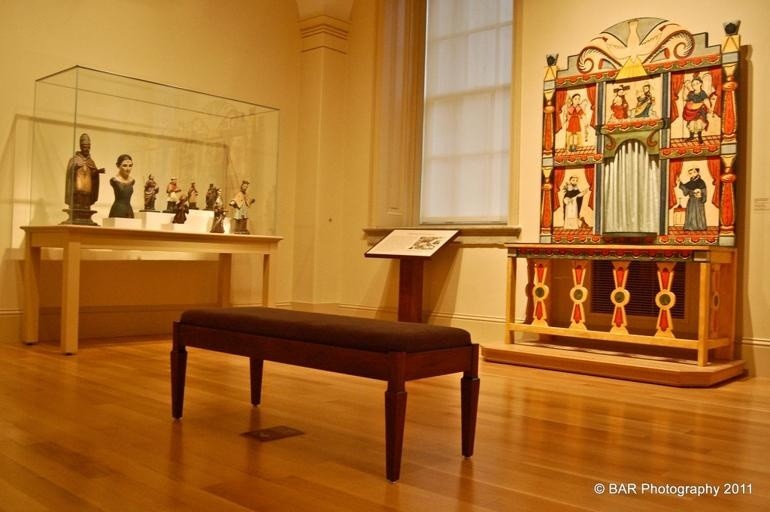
[20,225,285,355]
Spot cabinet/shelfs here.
[481,17,746,388]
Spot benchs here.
[170,306,480,482]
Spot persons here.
[109,154,136,217]
[562,173,582,232]
[634,86,653,118]
[143,173,226,233]
[64,132,105,210]
[562,91,590,154]
[684,75,708,143]
[676,166,708,231]
[610,86,630,120]
[229,179,255,234]
